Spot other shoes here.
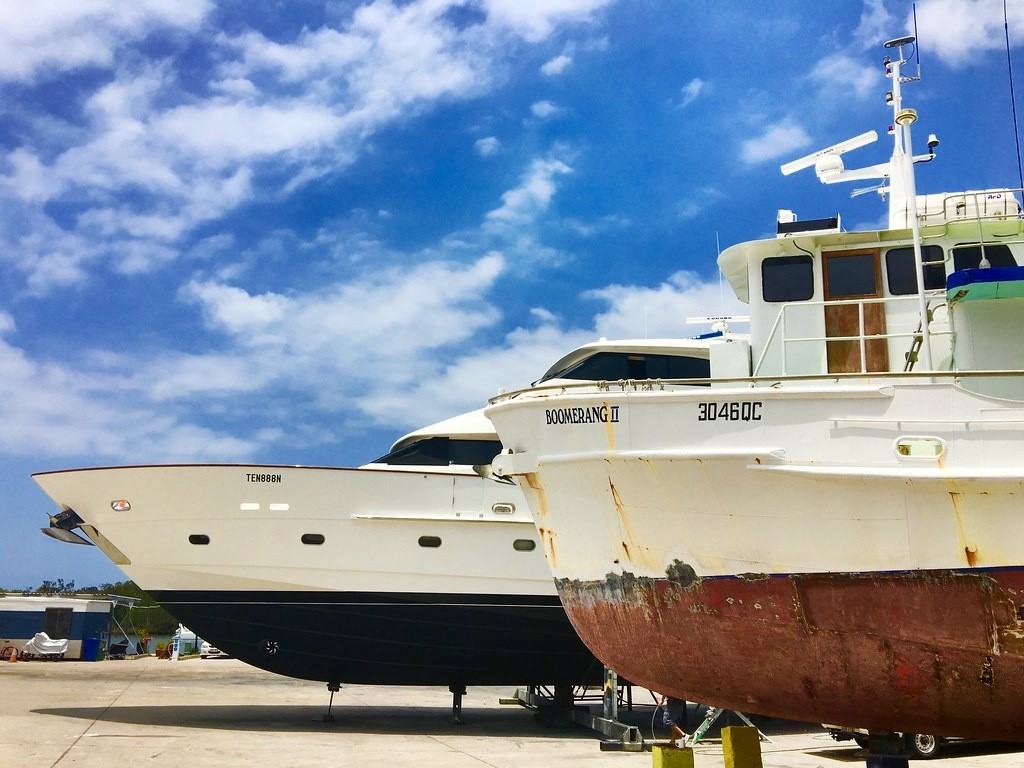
[682,733,690,743]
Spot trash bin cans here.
[83,637,99,661]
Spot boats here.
[483,38,1023,744]
[31,312,756,697]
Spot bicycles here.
[0,639,18,661]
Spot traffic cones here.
[7,645,18,663]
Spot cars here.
[200,641,229,659]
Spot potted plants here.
[156,642,166,657]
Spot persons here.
[657,695,689,746]
